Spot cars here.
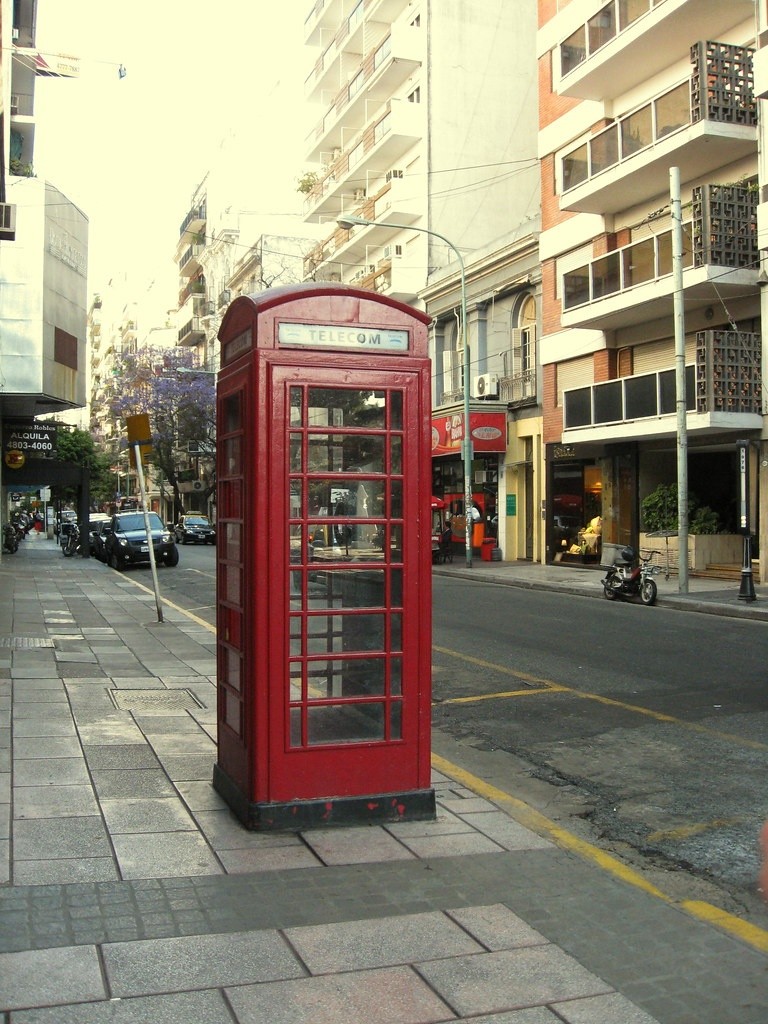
[173,510,216,545]
[53,510,112,562]
[553,515,583,539]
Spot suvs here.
[104,508,179,571]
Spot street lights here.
[333,208,477,568]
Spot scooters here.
[3,508,31,554]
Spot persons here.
[574,525,587,543]
[432,520,452,565]
[14,508,42,536]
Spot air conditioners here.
[474,373,497,397]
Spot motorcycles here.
[600,546,664,606]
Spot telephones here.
[334,458,383,548]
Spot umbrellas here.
[430,494,448,510]
